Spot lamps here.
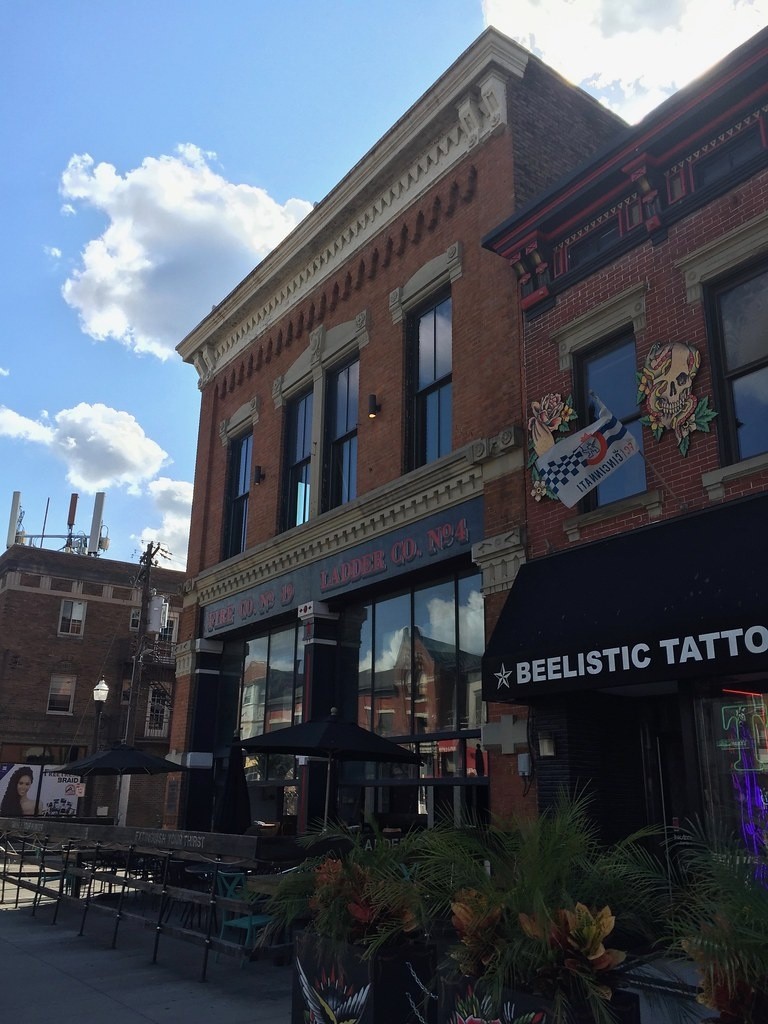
[254,465,265,486]
[367,394,382,418]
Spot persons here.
[0,767,44,816]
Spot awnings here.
[480,494,768,706]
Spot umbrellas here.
[226,706,427,833]
[211,729,253,837]
[41,741,189,826]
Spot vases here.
[291,929,437,1024]
[438,975,641,1024]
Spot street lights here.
[72,672,112,900]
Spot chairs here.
[61,851,233,933]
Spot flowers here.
[243,776,768,1024]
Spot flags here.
[534,401,639,508]
